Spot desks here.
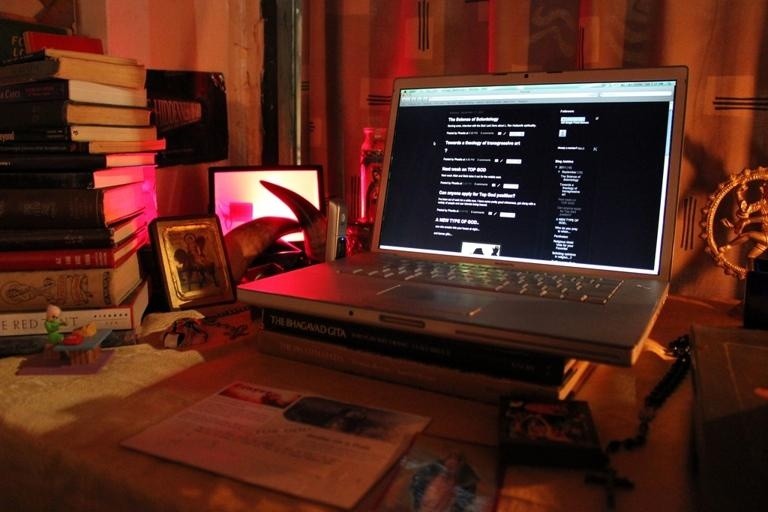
[0,265,766,510]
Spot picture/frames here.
[146,214,238,311]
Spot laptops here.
[237,65,689,367]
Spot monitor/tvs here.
[209,164,324,243]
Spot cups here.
[303,222,327,268]
